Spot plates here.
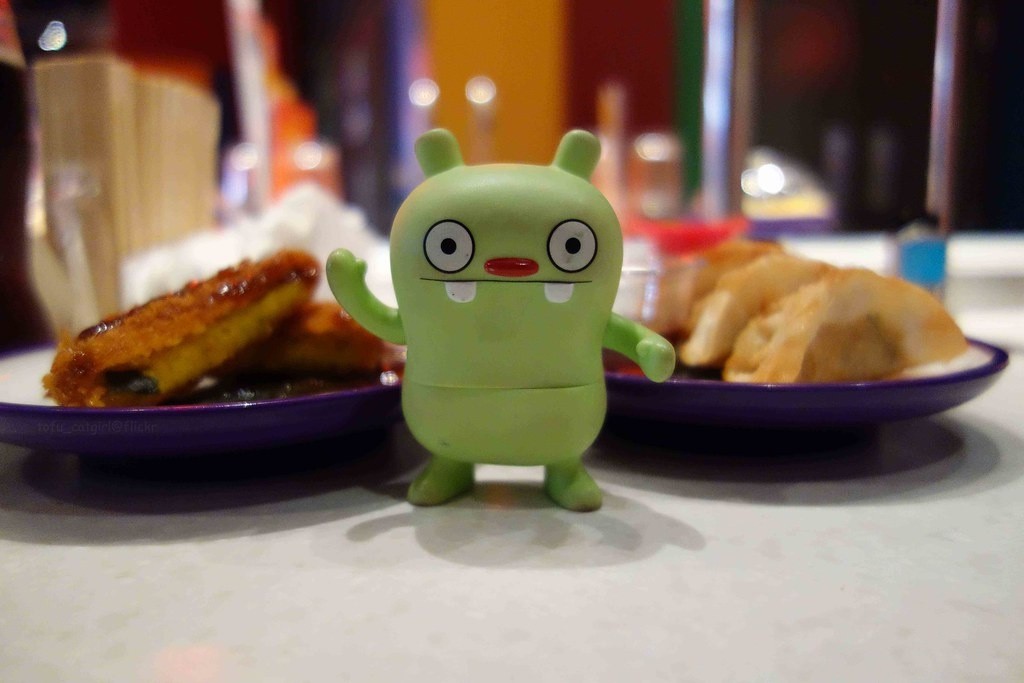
[0,343,401,478]
[589,321,1014,469]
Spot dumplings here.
[649,236,970,383]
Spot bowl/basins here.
[609,271,650,320]
